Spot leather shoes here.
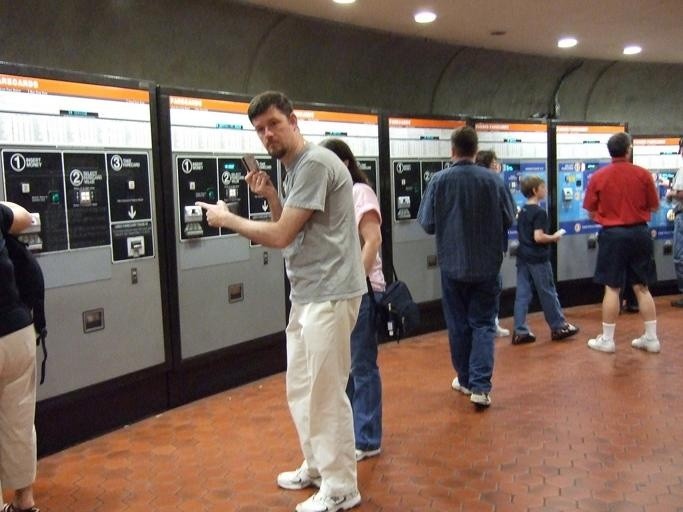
[670,298,683,306]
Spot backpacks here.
[1,205,49,385]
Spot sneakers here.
[494,316,510,337]
[451,377,472,397]
[277,471,329,490]
[513,330,537,344]
[631,334,660,352]
[587,334,616,353]
[551,323,579,341]
[470,387,492,405]
[355,442,381,462]
[1,503,41,512]
[294,486,362,512]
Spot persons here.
[195,90,369,512]
[476,151,510,338]
[316,138,389,463]
[657,137,683,308]
[418,126,516,406]
[582,133,662,354]
[0,198,39,512]
[512,178,580,345]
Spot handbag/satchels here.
[364,241,421,343]
